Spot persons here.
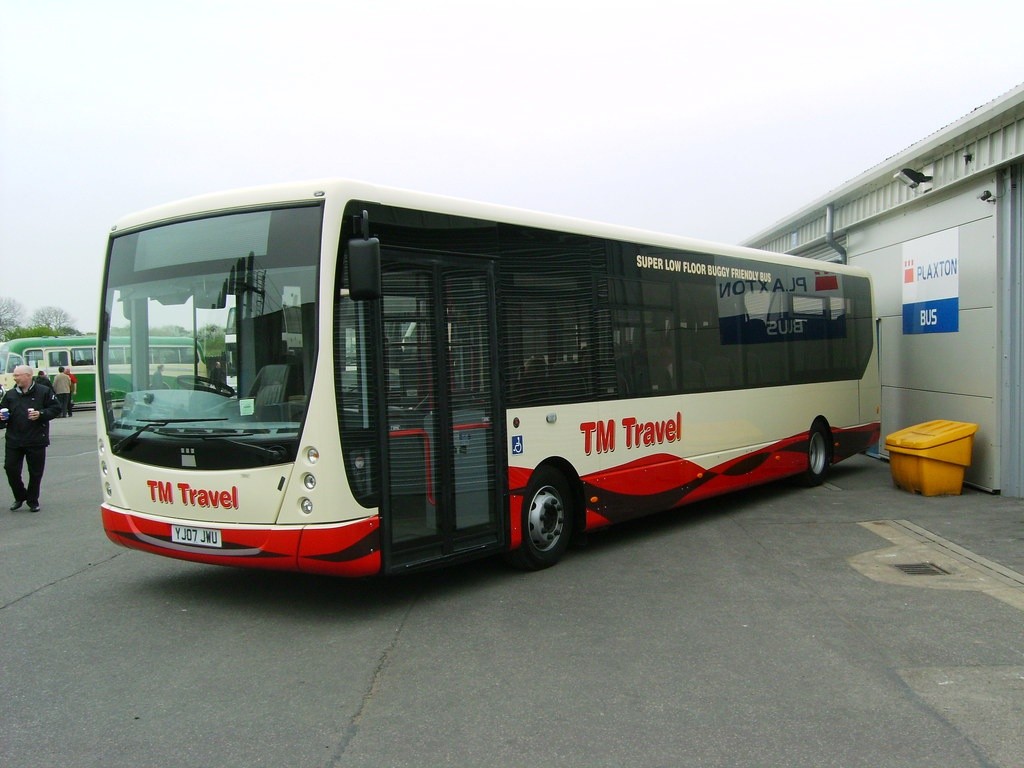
[36,366,78,418]
[150,365,164,389]
[0,365,63,511]
[210,360,226,392]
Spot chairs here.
[245,364,296,406]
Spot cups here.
[28,408,34,419]
[0,408,9,421]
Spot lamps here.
[893,168,932,189]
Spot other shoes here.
[9,499,25,510]
[26,502,40,512]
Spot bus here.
[0,335,210,412]
[223,305,306,400]
[94,181,880,582]
